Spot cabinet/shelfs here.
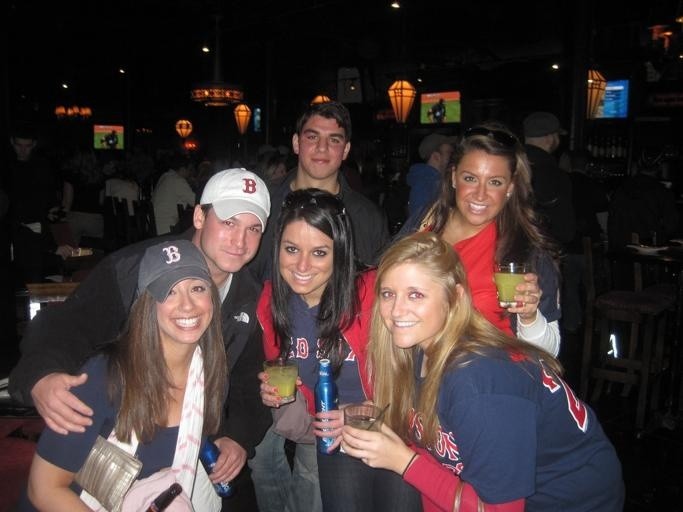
[626,51,682,179]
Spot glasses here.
[459,124,517,148]
[284,190,345,215]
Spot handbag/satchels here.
[73,434,144,512]
[78,344,206,512]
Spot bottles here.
[200,439,233,497]
[314,358,337,456]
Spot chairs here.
[103,194,193,249]
[582,237,671,434]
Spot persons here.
[255,187,424,512]
[122,144,287,236]
[525,111,577,244]
[16,240,230,512]
[3,125,74,285]
[426,98,446,124]
[248,102,391,512]
[608,159,675,287]
[394,124,561,357]
[405,133,453,217]
[340,233,626,511]
[9,168,273,512]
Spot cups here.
[262,359,298,406]
[493,262,528,309]
[343,406,383,434]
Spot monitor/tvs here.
[415,87,465,127]
[90,120,129,153]
[583,76,635,125]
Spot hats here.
[136,239,213,305]
[522,112,568,138]
[418,134,455,161]
[200,167,272,233]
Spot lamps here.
[646,0,682,60]
[387,80,416,127]
[189,33,244,108]
[53,103,92,119]
[234,104,252,135]
[308,93,334,108]
[173,119,192,141]
